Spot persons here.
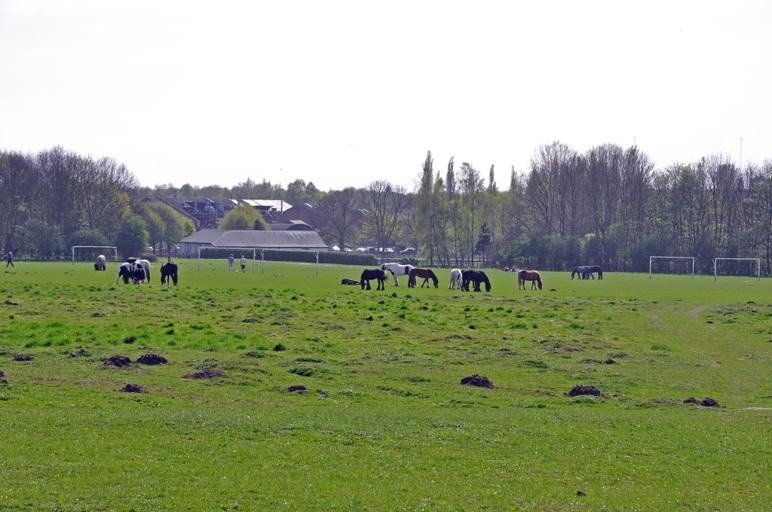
[229,253,234,271]
[5,248,16,267]
[503,263,518,272]
[240,255,250,272]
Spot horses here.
[94,254,106,271]
[408,268,438,288]
[342,279,359,284]
[448,269,491,291]
[161,263,177,285]
[518,270,542,290]
[381,262,416,286]
[114,257,151,284]
[361,268,387,290]
[572,265,603,280]
